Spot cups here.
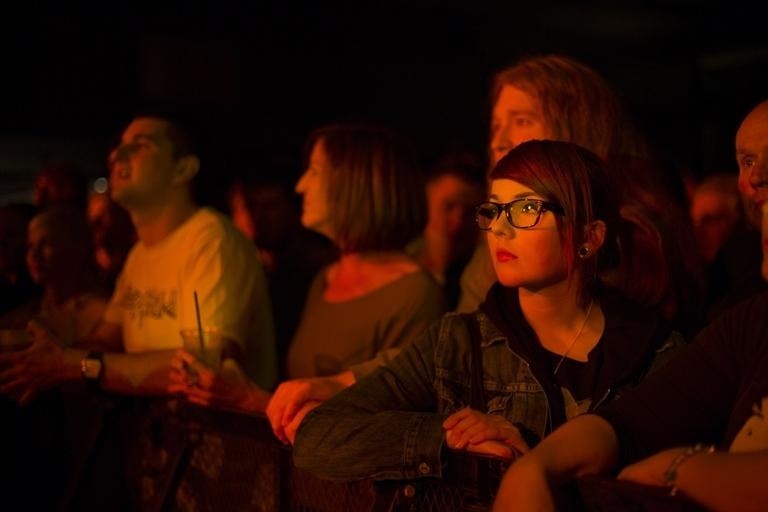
[179,327,226,392]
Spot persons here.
[0,54,766,511]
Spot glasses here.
[473,198,565,231]
